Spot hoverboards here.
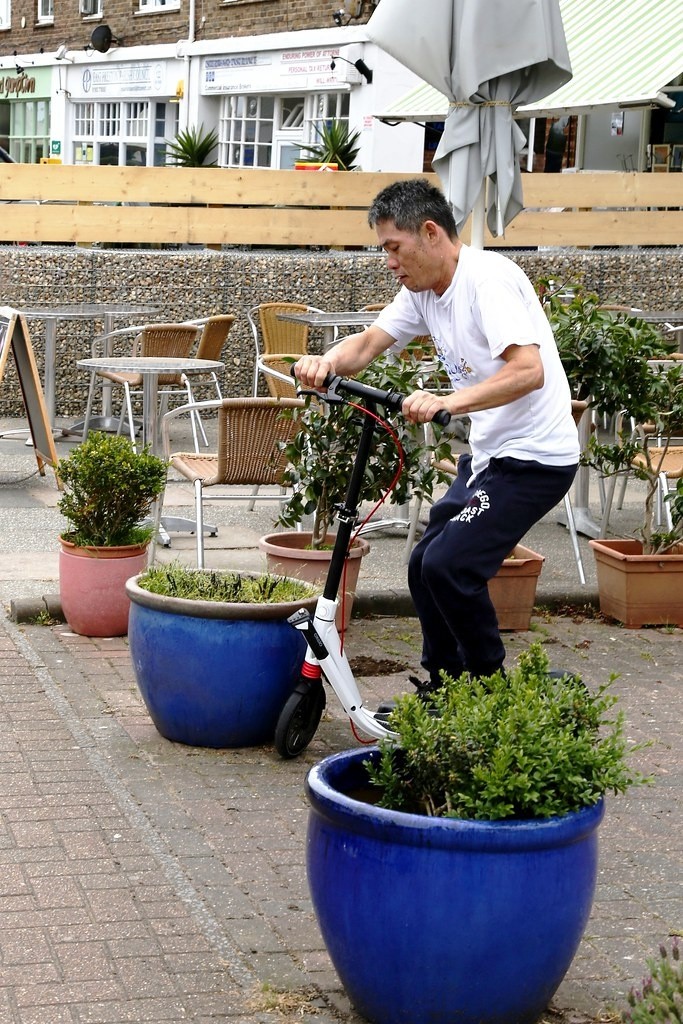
[273,359,590,763]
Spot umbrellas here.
[363,1,572,249]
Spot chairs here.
[81,323,199,454]
[147,398,321,570]
[157,315,235,447]
[247,303,683,587]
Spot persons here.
[292,179,580,738]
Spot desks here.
[620,306,681,333]
[275,311,381,417]
[77,357,225,548]
[13,305,164,448]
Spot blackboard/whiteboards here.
[0,307,59,468]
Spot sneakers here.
[377,675,449,712]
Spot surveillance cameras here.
[55,44,67,60]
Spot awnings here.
[371,1,682,130]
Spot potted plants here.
[486,271,683,633]
[304,637,657,1024]
[125,558,327,749]
[259,340,455,633]
[51,428,172,638]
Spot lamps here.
[14,60,35,74]
